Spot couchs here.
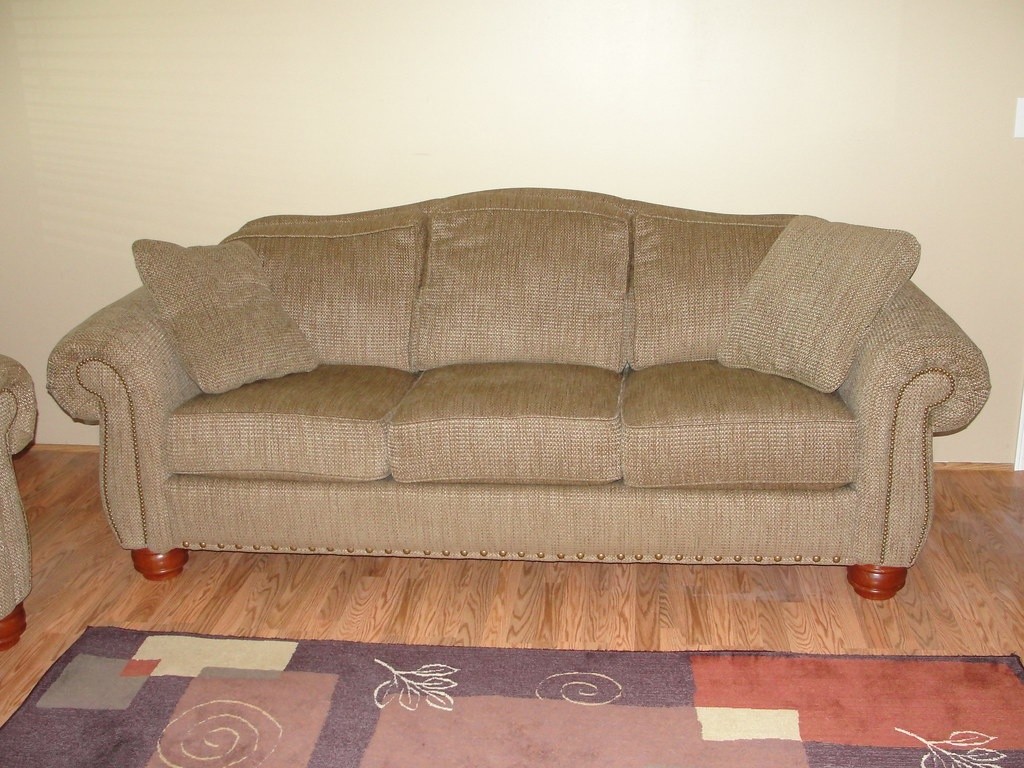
[0,353,38,650]
[46,185,990,600]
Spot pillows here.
[716,216,922,394]
[130,240,318,397]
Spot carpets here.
[0,624,1024,768]
